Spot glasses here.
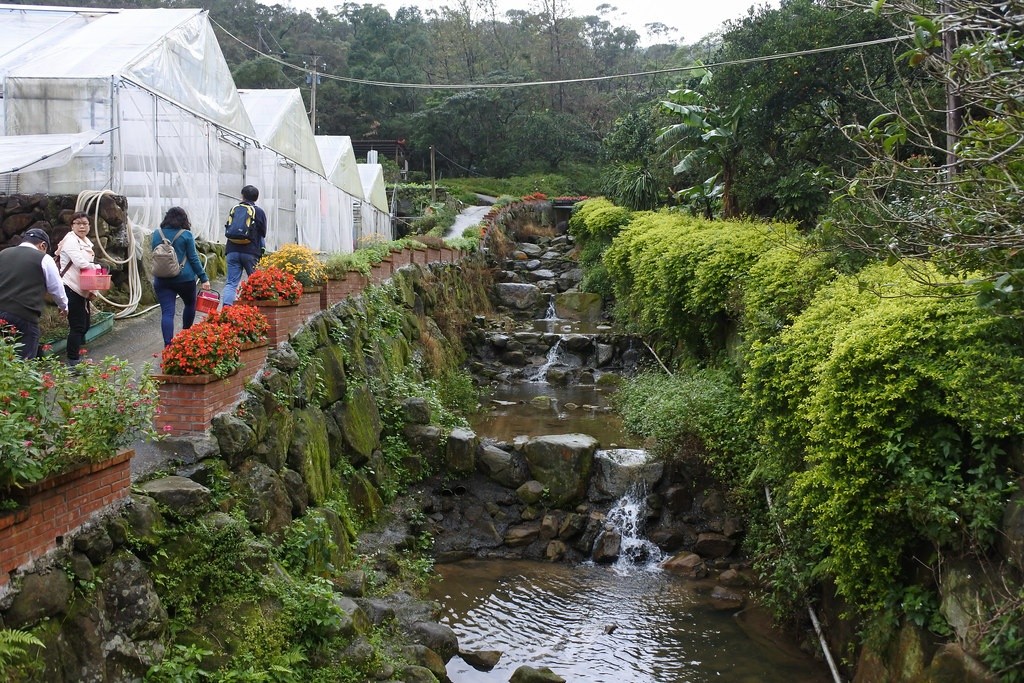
[72,221,89,227]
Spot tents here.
[0,4,392,259]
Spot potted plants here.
[321,194,524,310]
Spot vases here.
[0,447,136,590]
[300,286,324,325]
[237,337,273,396]
[232,298,302,352]
[524,199,582,206]
[85,310,115,344]
[38,335,66,361]
[148,365,240,437]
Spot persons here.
[222,185,267,308]
[54,211,103,367]
[151,206,210,348]
[0,228,69,361]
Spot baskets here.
[196,287,220,314]
[80,261,113,290]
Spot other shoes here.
[69,359,86,365]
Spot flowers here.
[252,240,329,288]
[523,191,589,201]
[0,302,272,515]
[235,264,306,305]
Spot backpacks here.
[151,229,187,277]
[225,203,260,245]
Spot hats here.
[26,229,51,254]
